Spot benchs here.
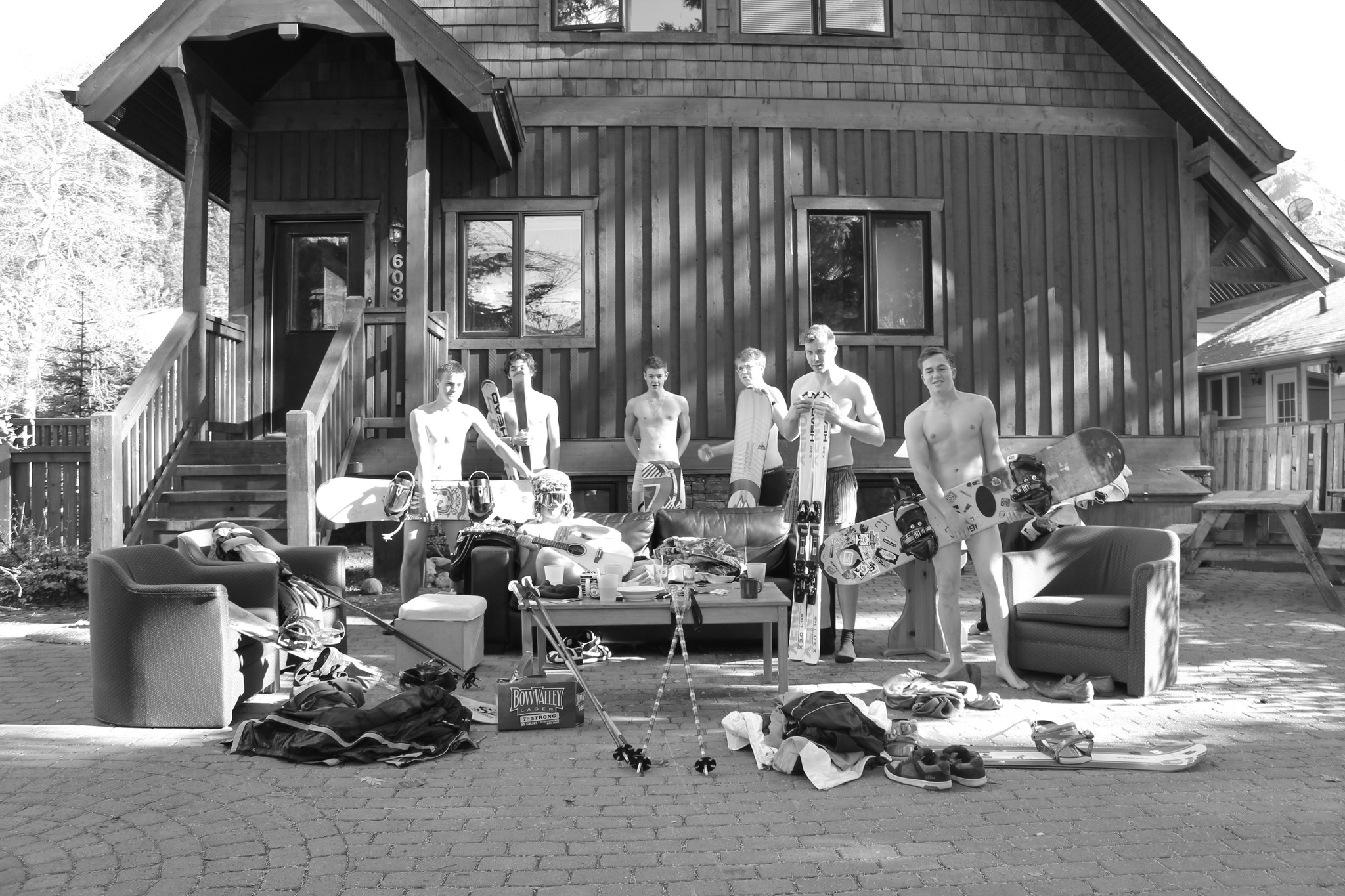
[1159,523,1198,547]
[1317,527,1344,566]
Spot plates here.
[616,586,665,601]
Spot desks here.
[877,542,978,662]
[1176,488,1344,615]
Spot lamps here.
[387,214,406,246]
[1248,367,1263,386]
[1326,355,1343,375]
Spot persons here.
[623,358,692,515]
[903,347,1031,693]
[475,351,561,480]
[516,469,651,599]
[382,360,533,635]
[694,346,791,504]
[781,318,887,667]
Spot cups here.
[747,562,767,590]
[597,574,618,602]
[740,578,762,599]
[653,564,668,592]
[683,568,697,592]
[604,564,624,588]
[544,565,565,586]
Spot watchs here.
[771,398,778,406]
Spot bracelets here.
[509,434,515,448]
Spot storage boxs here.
[496,673,585,732]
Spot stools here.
[391,592,487,682]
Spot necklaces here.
[565,516,572,526]
[930,392,957,416]
[646,389,665,417]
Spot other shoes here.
[382,613,401,636]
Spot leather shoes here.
[1073,672,1116,698]
[1033,675,1095,703]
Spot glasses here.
[735,364,758,373]
[534,491,569,508]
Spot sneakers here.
[935,744,988,787]
[884,748,953,790]
[546,636,584,670]
[578,630,612,664]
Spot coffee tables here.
[516,579,791,698]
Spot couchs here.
[470,505,799,653]
[178,526,349,695]
[86,541,280,731]
[999,524,1182,700]
